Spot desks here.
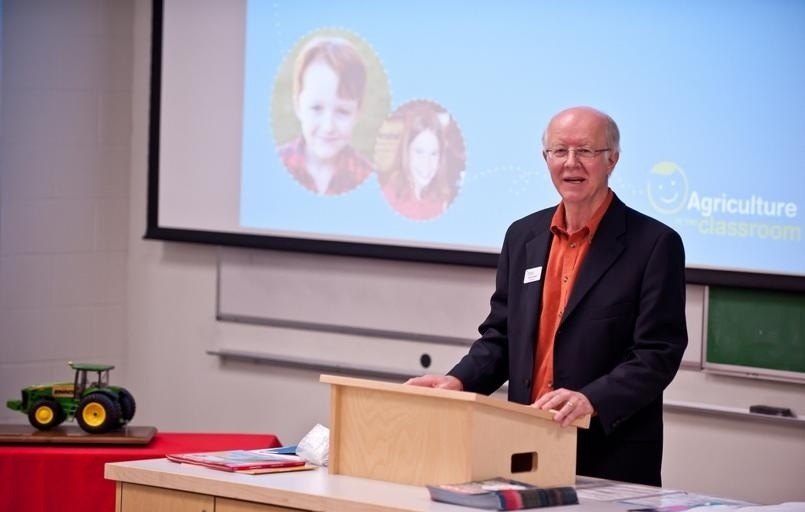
[101,445,655,512]
[0,430,282,512]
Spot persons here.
[278,35,374,194]
[379,107,458,219]
[401,105,689,488]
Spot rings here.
[566,402,576,409]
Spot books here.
[163,449,319,475]
[426,475,580,512]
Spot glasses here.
[545,144,614,159]
